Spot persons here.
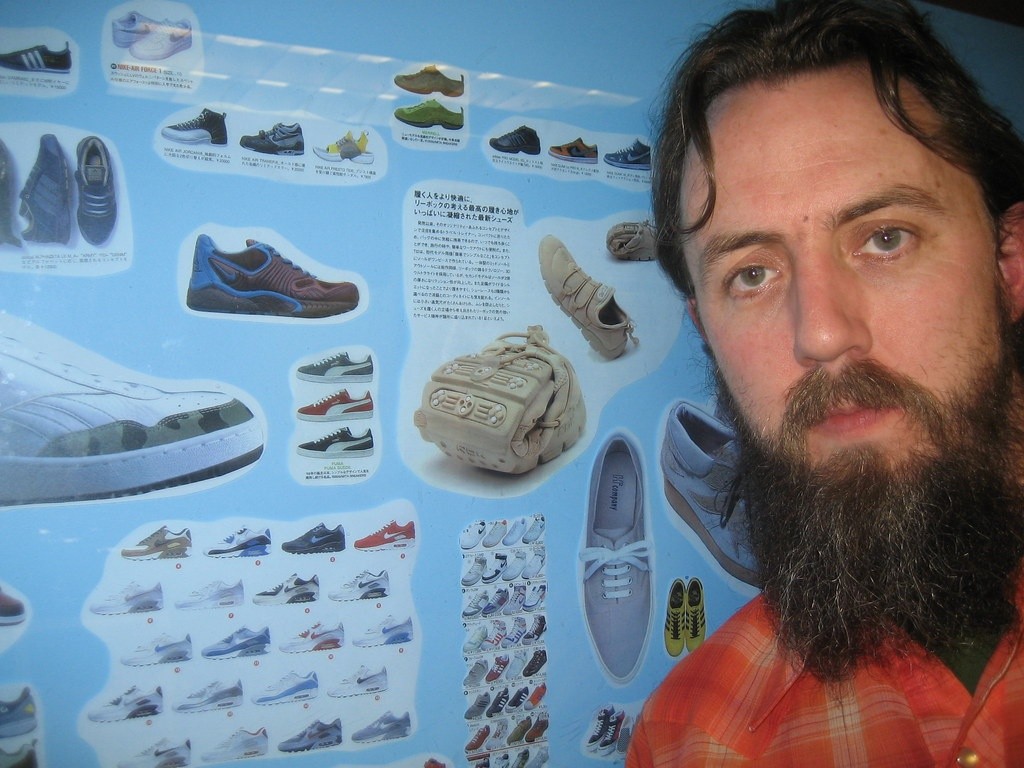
[625,1,1023,767]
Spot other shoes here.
[0,588,27,626]
[0,687,37,739]
[0,139,22,248]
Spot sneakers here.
[19,134,71,244]
[606,219,658,261]
[87,520,452,768]
[660,399,769,590]
[161,108,227,147]
[0,41,72,65]
[586,703,634,756]
[296,388,373,422]
[112,11,192,61]
[459,512,550,768]
[394,65,464,97]
[489,125,541,155]
[414,325,586,475]
[239,122,304,156]
[186,234,359,319]
[663,577,707,657]
[74,136,117,246]
[296,351,373,384]
[576,427,656,686]
[296,427,374,459]
[0,739,39,768]
[538,234,639,360]
[548,137,598,164]
[0,310,267,512]
[603,138,651,171]
[394,99,464,130]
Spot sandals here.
[313,130,374,164]
